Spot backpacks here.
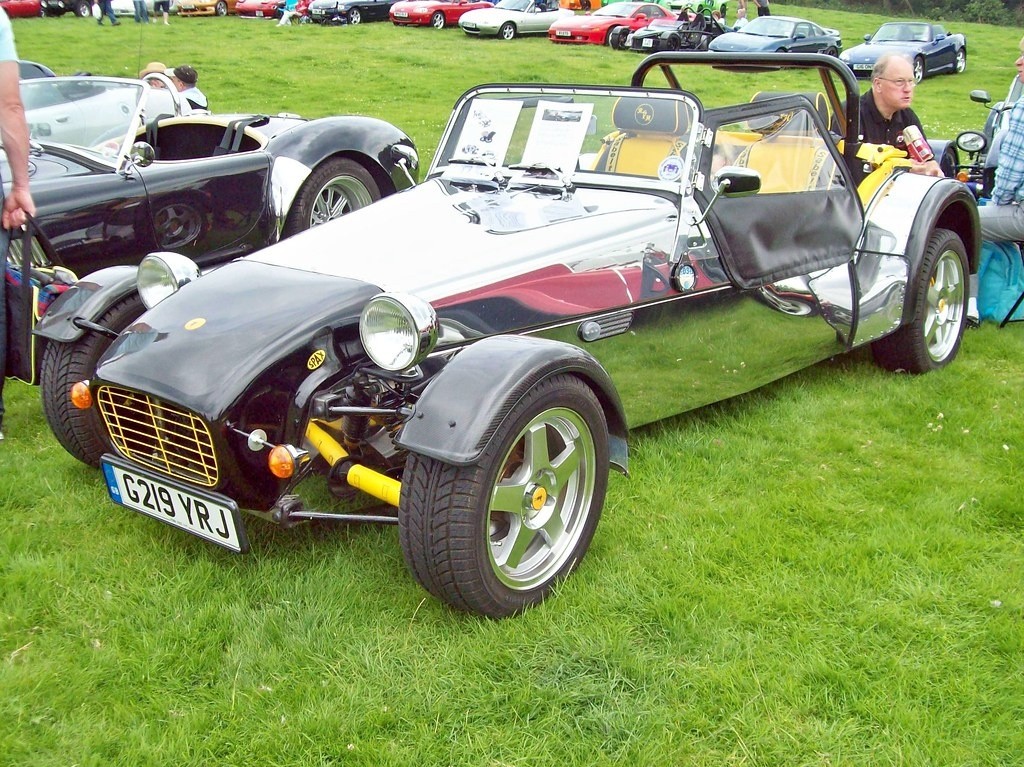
[975,240,1024,320]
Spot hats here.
[140,62,166,77]
[164,65,198,83]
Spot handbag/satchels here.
[6,211,71,386]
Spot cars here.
[390,0,495,29]
[548,0,734,54]
[838,22,967,84]
[72,55,1024,621]
[458,0,575,40]
[0,0,393,26]
[0,63,418,281]
[708,16,843,58]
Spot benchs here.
[589,129,911,204]
[135,121,262,161]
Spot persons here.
[965,36,1024,330]
[133,0,149,24]
[94,0,121,26]
[733,9,748,27]
[831,50,926,159]
[737,0,748,10]
[753,0,770,16]
[153,0,170,25]
[712,10,724,25]
[276,0,298,26]
[0,5,36,439]
[140,62,209,113]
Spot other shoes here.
[163,21,169,25]
[97,22,104,26]
[112,22,121,26]
[153,18,157,24]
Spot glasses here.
[878,77,914,86]
